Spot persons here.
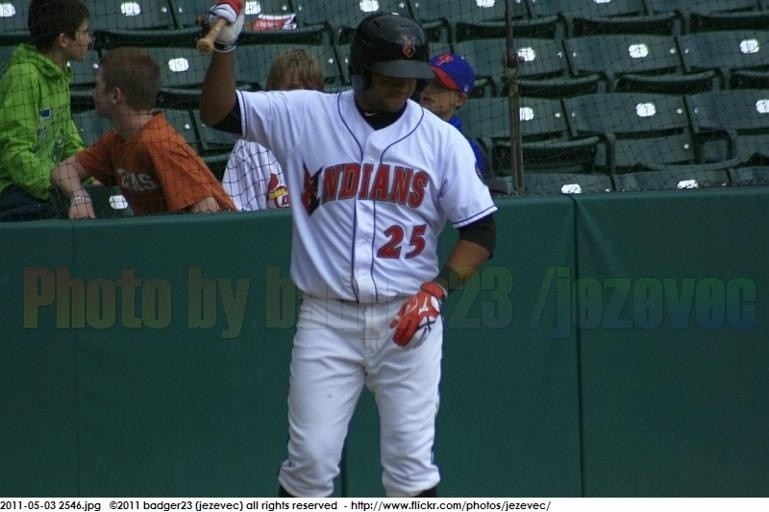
[51,43,239,222]
[418,53,494,197]
[0,0,93,222]
[220,48,335,214]
[199,2,497,496]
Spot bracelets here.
[69,194,93,203]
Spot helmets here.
[347,12,436,92]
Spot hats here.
[427,52,475,92]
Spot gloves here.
[390,281,446,352]
[202,0,241,54]
